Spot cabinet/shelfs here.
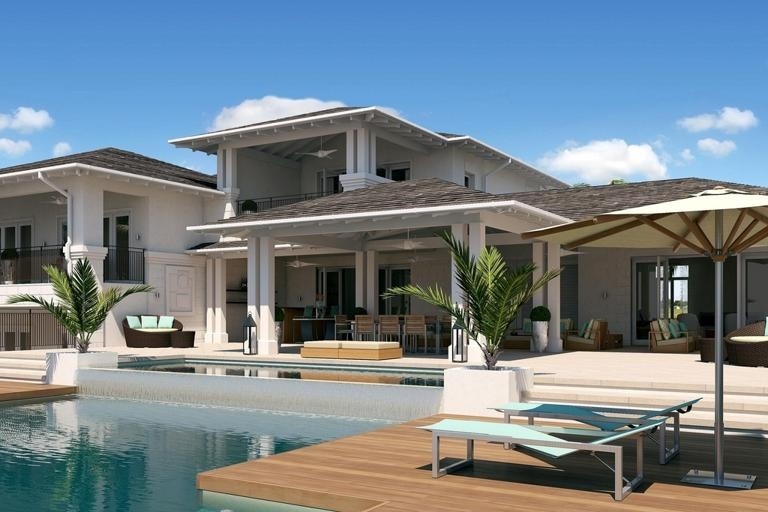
[225,288,279,306]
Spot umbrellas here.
[520,185,768,490]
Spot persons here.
[314,293,327,340]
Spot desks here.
[171,330,194,348]
[291,316,336,341]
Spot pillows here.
[126,315,141,328]
[157,316,175,329]
[140,316,157,328]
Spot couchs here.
[122,314,197,348]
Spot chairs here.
[413,416,669,503]
[500,317,625,353]
[332,312,453,356]
[488,395,703,464]
[648,312,768,367]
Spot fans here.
[40,192,69,206]
[283,255,321,269]
[293,135,340,162]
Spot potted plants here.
[0,247,18,285]
[2,257,160,388]
[381,229,566,418]
